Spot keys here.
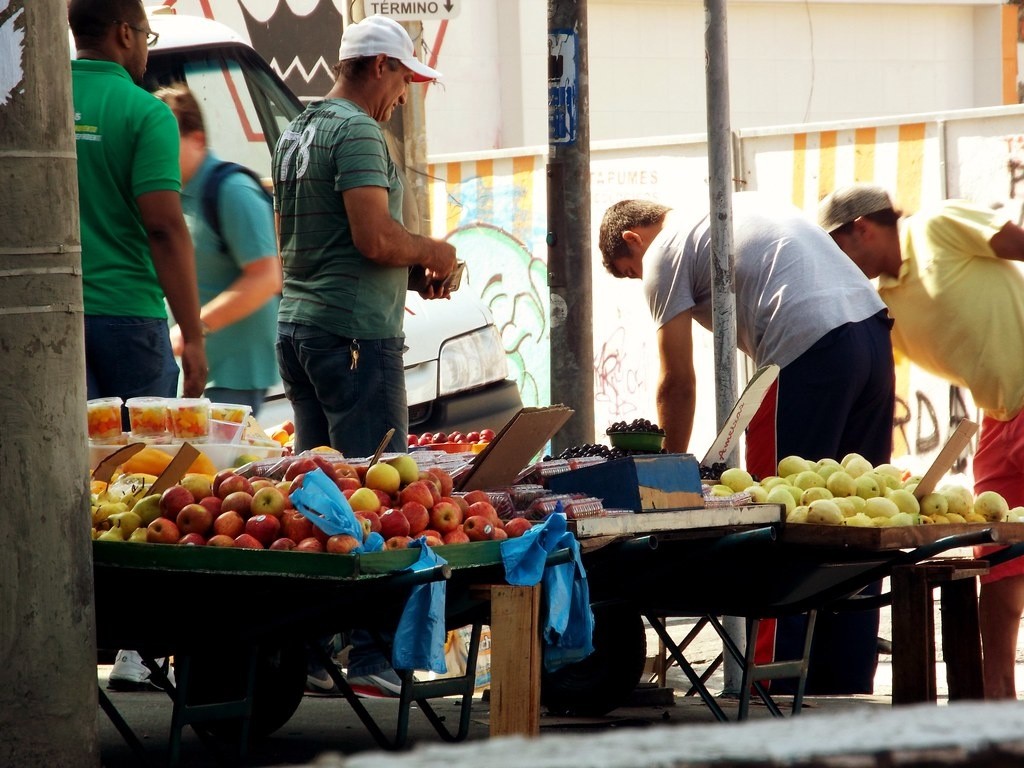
[350,350,359,369]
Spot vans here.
[1,0,526,446]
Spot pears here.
[90,474,216,544]
[707,452,1024,527]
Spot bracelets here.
[200,320,210,337]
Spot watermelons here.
[87,406,240,440]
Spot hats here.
[338,14,442,83]
[813,183,893,233]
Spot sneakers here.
[106,648,176,692]
[346,668,420,698]
[303,659,347,698]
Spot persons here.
[150,87,283,416]
[812,184,1024,702]
[598,190,895,696]
[69,0,210,691]
[271,13,458,702]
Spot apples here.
[408,429,497,446]
[270,421,295,457]
[145,456,533,553]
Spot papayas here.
[89,447,264,494]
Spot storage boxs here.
[89,440,289,475]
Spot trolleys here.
[534,526,1024,722]
[92,526,573,754]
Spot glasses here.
[113,20,159,45]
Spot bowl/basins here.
[87,394,254,449]
[607,431,666,453]
